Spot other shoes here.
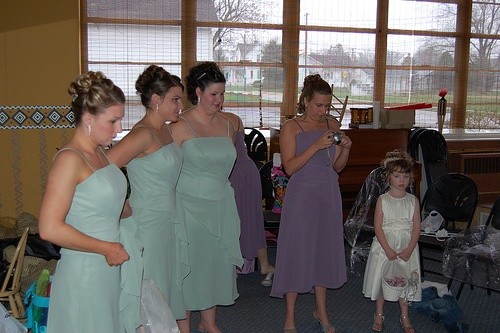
[258,265,276,286]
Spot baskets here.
[381,257,409,302]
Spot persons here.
[38,71,133,333]
[169,61,241,333]
[372,157,422,333]
[100,64,188,333]
[218,94,274,275]
[279,73,351,333]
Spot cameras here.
[330,132,341,145]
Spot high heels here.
[284,328,296,333]
[312,312,336,333]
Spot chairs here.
[0,228,30,318]
[241,125,291,228]
[343,128,500,300]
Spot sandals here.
[400,315,415,333]
[372,313,385,331]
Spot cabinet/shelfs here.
[269,123,421,219]
